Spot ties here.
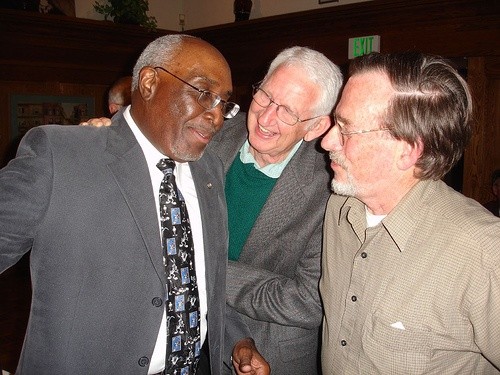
[156,157,201,375]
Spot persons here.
[0,34,272,375]
[319,49,500,375]
[108,75,133,116]
[79,46,343,375]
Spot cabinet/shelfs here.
[445,54,500,205]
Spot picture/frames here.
[9,94,96,155]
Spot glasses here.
[334,112,393,146]
[252,80,325,125]
[154,66,240,119]
